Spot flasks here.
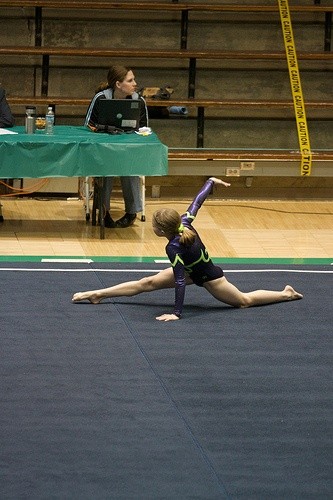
[25,105,36,134]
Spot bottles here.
[46,107,54,135]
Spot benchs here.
[0,0,333,197]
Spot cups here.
[36,117,45,129]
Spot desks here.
[0,123,169,240]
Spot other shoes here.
[116,213,136,227]
[98,211,116,227]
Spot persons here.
[84,65,148,228]
[72,177,304,321]
[0,88,15,222]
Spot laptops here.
[99,99,141,132]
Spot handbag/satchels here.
[136,85,174,120]
